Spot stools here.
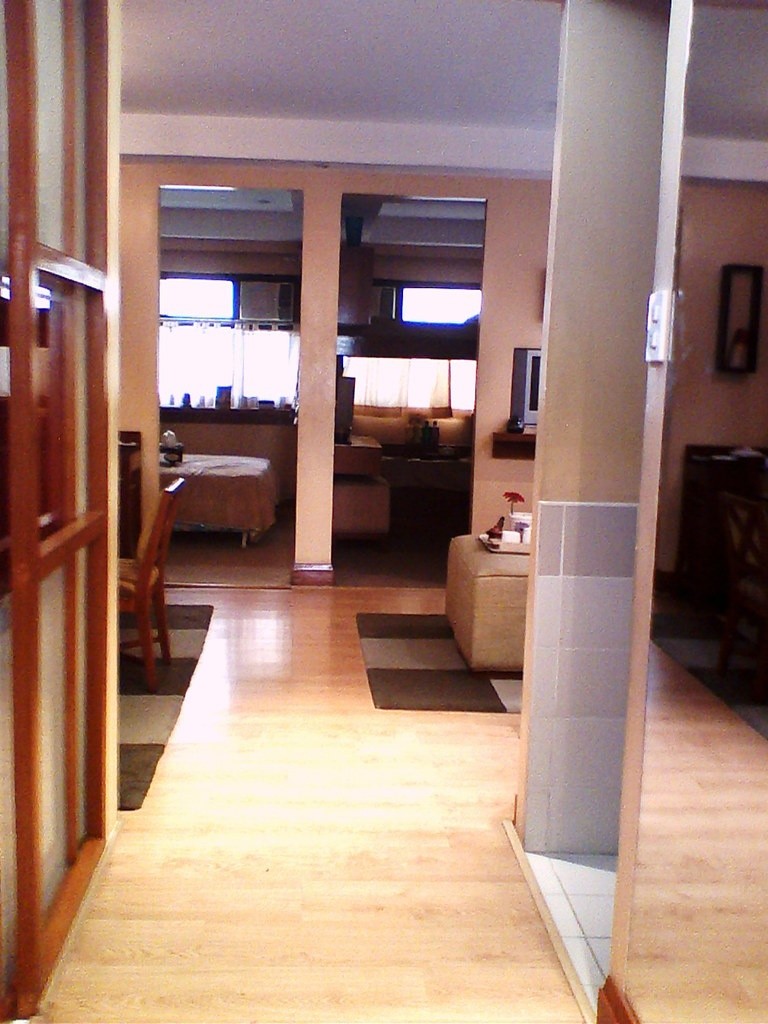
[446,534,531,673]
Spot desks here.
[672,443,768,612]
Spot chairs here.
[714,490,768,703]
[113,478,186,676]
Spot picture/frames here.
[716,265,764,372]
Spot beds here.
[158,453,278,549]
